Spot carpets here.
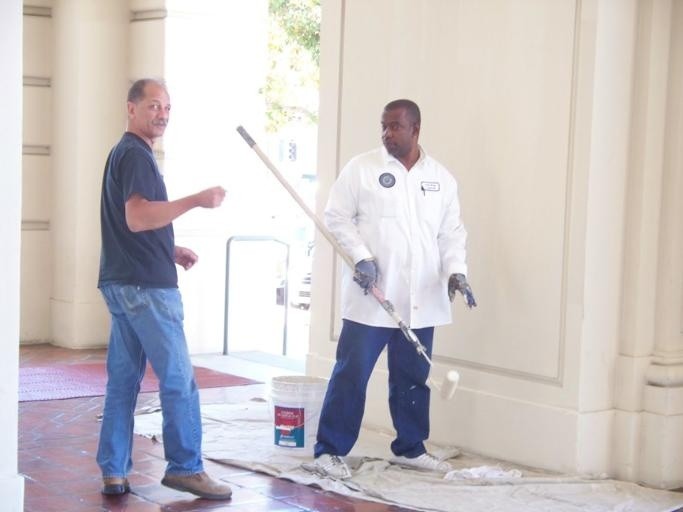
[100,397,683,512]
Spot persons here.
[96,78,233,501]
[310,98,477,481]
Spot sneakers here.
[388,451,451,472]
[301,453,351,479]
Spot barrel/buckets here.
[268,374,330,455]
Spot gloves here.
[448,273,477,309]
[352,259,377,296]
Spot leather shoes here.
[101,477,129,494]
[161,466,232,499]
